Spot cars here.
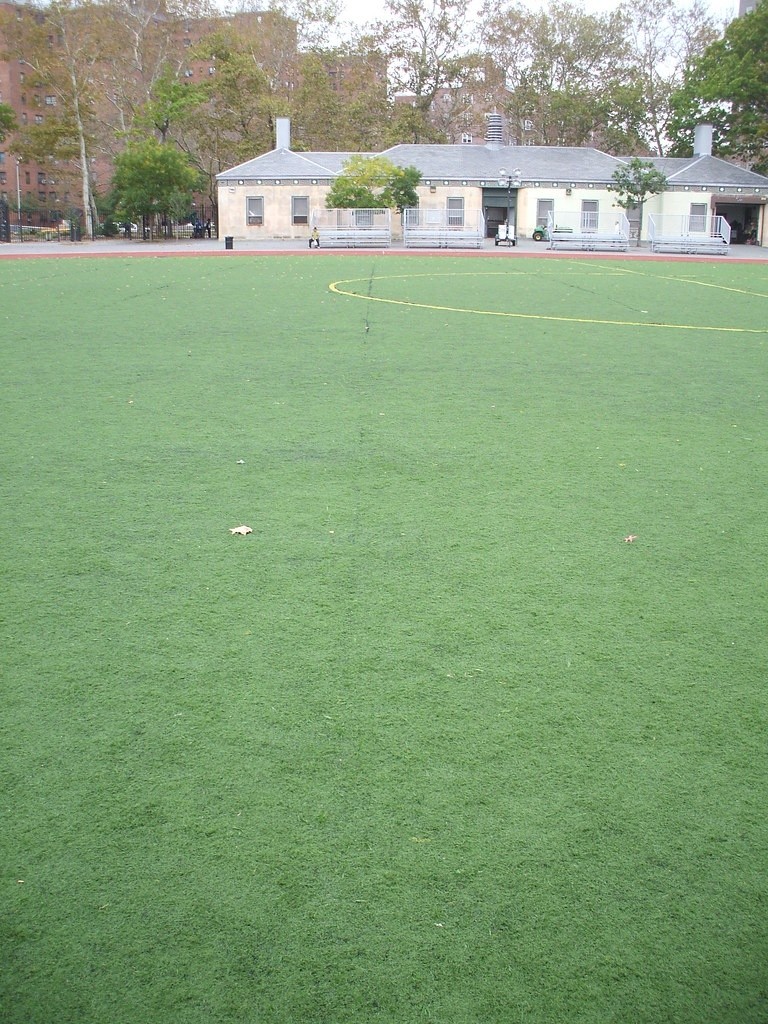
[111,221,150,234]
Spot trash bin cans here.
[225,236,234,249]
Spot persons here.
[309,227,320,249]
[124,218,131,238]
[194,217,212,239]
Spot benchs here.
[312,228,391,248]
[405,230,485,248]
[550,230,628,253]
[651,233,728,255]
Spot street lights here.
[16,156,23,224]
